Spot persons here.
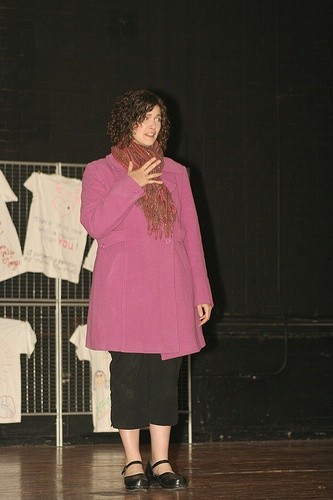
[79,88,214,494]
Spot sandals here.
[144,460,188,489]
[121,461,148,489]
